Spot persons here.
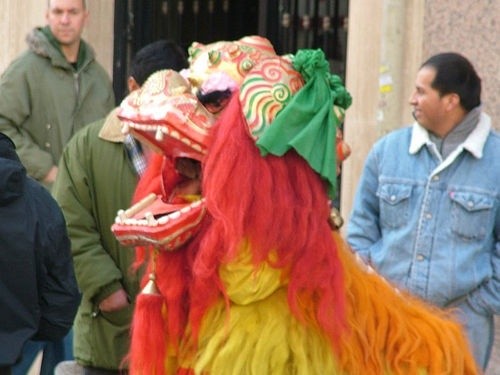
[0,133,80,375]
[0,0,116,196]
[346,52,500,375]
[49,40,195,375]
